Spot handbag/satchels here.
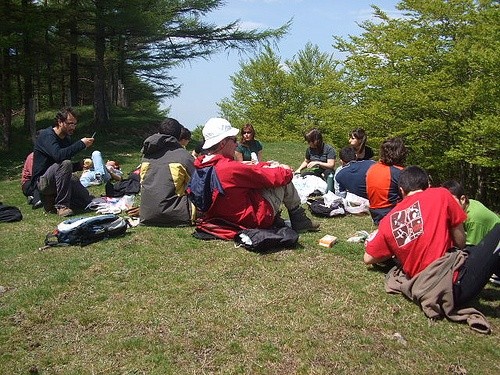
[233,226,299,253]
[105,176,141,197]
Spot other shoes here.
[32,200,42,210]
[57,207,73,216]
[27,196,34,204]
[0,202,22,223]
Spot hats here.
[202,117,240,150]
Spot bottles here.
[251,151,259,165]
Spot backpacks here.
[44,211,133,246]
[306,198,346,217]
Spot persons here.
[21,151,42,208]
[80,151,123,187]
[293,129,334,196]
[139,117,196,228]
[32,107,99,217]
[366,138,408,225]
[348,128,375,161]
[335,147,375,199]
[442,179,500,246]
[185,118,320,233]
[363,166,500,311]
[179,123,263,163]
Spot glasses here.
[65,121,77,128]
[242,131,252,134]
[227,137,237,143]
